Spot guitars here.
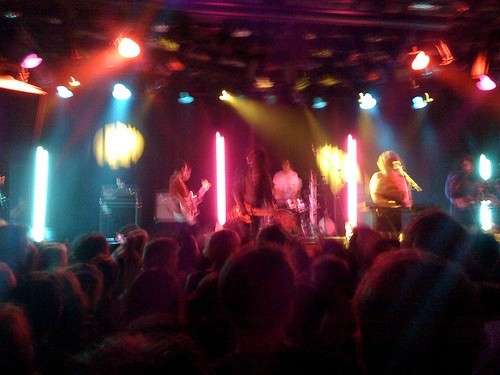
[179,182,212,224]
[231,202,275,224]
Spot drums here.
[284,195,309,215]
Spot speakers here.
[99,201,141,244]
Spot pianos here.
[359,200,439,214]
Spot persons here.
[99,173,139,238]
[369,151,412,243]
[232,146,278,244]
[0,172,19,227]
[168,160,199,234]
[300,166,328,236]
[272,159,304,237]
[481,176,500,229]
[444,153,489,232]
[0,220,500,375]
